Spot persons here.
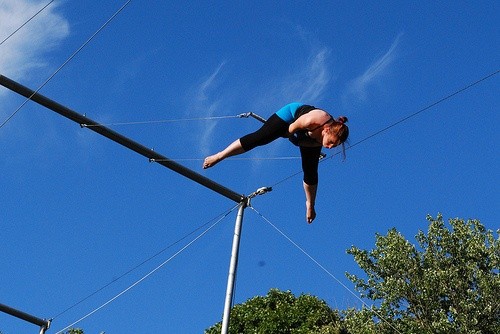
[202,101,349,224]
